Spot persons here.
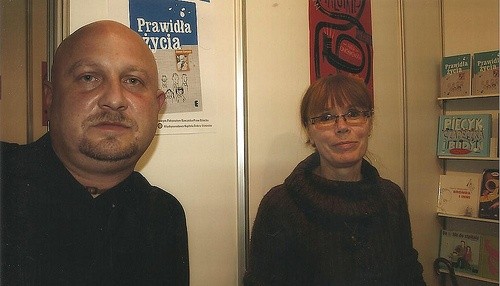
[243,75,426,286]
[0,20,190,286]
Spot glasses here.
[305,110,375,130]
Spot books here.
[439,230,480,275]
[479,169,499,221]
[437,113,500,158]
[478,233,499,282]
[442,54,473,97]
[473,50,500,96]
[439,274,499,286]
[437,175,480,217]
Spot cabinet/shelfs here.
[437,93,500,285]
